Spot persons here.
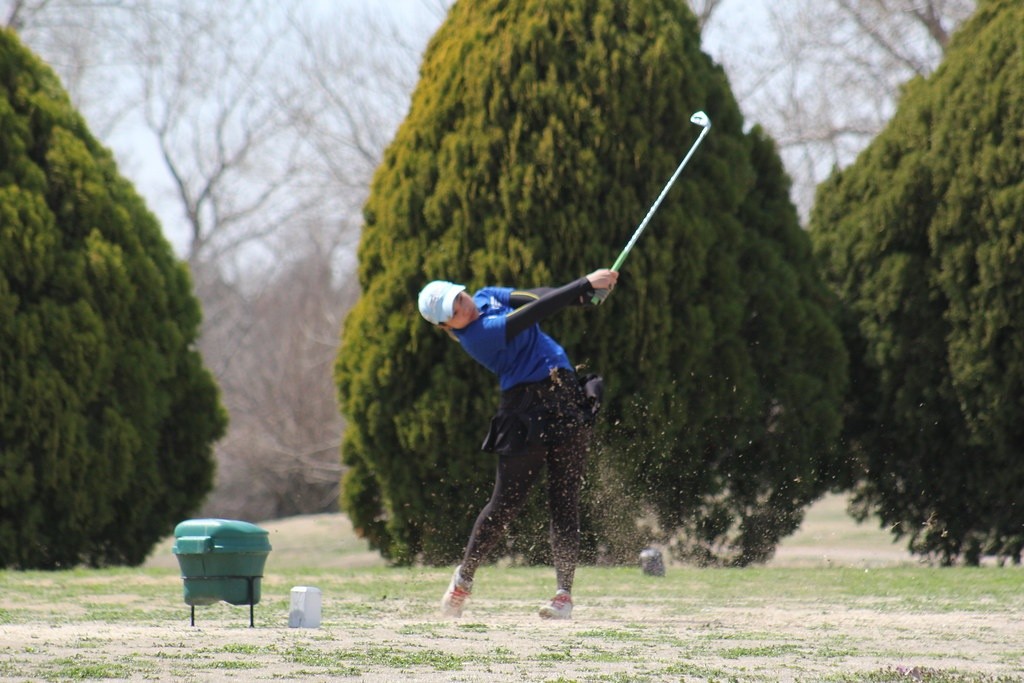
[418,268,618,621]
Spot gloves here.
[595,284,613,305]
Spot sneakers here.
[539,590,573,620]
[444,566,475,620]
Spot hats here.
[418,281,466,324]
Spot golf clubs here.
[590,111,713,306]
